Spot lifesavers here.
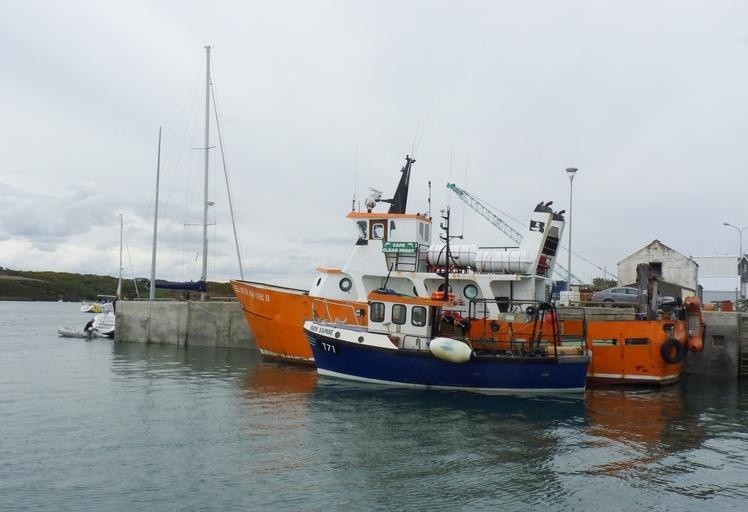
[431,291,454,301]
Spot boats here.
[299,208,591,398]
[228,154,707,390]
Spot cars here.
[589,287,662,307]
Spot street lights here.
[722,223,748,312]
[566,165,580,295]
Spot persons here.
[82,318,96,338]
